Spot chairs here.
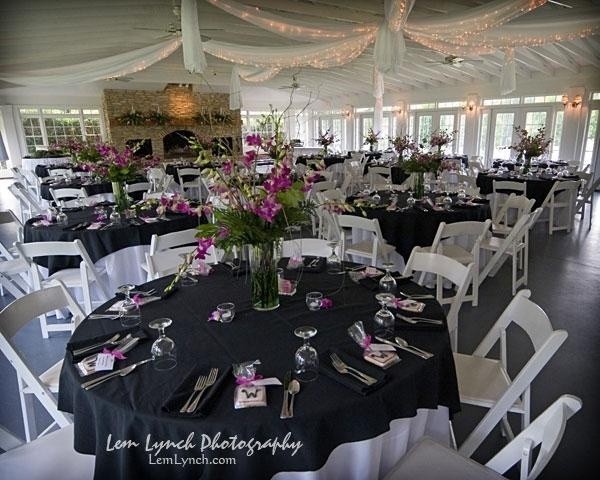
[384,433,512,479]
[483,392,582,480]
[1,424,99,479]
[0,126,600,353]
[1,278,93,440]
[448,289,570,449]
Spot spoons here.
[87,315,122,322]
[86,364,136,393]
[70,222,88,231]
[395,335,436,359]
[288,378,302,418]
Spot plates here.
[225,260,240,271]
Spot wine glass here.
[326,242,341,272]
[489,157,570,179]
[45,191,166,225]
[147,317,178,371]
[54,167,102,185]
[294,324,320,381]
[373,291,396,329]
[118,282,140,328]
[178,252,198,287]
[362,177,465,209]
[379,263,397,291]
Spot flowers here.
[164,55,334,303]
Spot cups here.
[304,292,324,312]
[217,302,235,324]
[276,268,284,279]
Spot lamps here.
[391,105,407,114]
[460,101,474,116]
[560,93,583,109]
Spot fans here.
[422,56,486,69]
[133,6,214,45]
[278,72,309,92]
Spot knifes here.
[394,273,416,280]
[279,369,291,418]
[374,334,428,362]
[100,222,115,232]
[80,355,152,388]
[87,314,143,317]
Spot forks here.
[177,374,207,414]
[306,258,320,269]
[72,331,135,356]
[114,287,159,297]
[399,291,434,300]
[187,366,218,415]
[329,352,378,388]
[395,312,443,326]
